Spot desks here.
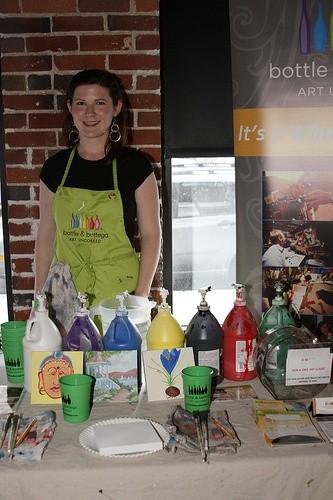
[0,375,333,500]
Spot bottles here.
[256,324,327,400]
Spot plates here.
[78,417,171,458]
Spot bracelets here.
[32,300,46,308]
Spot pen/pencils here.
[14,418,38,449]
[208,415,234,441]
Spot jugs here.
[88,295,159,350]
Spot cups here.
[180,365,214,414]
[58,374,93,423]
[0,321,27,382]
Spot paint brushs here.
[192,408,211,463]
[0,410,24,461]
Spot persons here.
[263,171,333,341]
[29,68,162,312]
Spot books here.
[251,399,326,448]
[31,347,195,404]
